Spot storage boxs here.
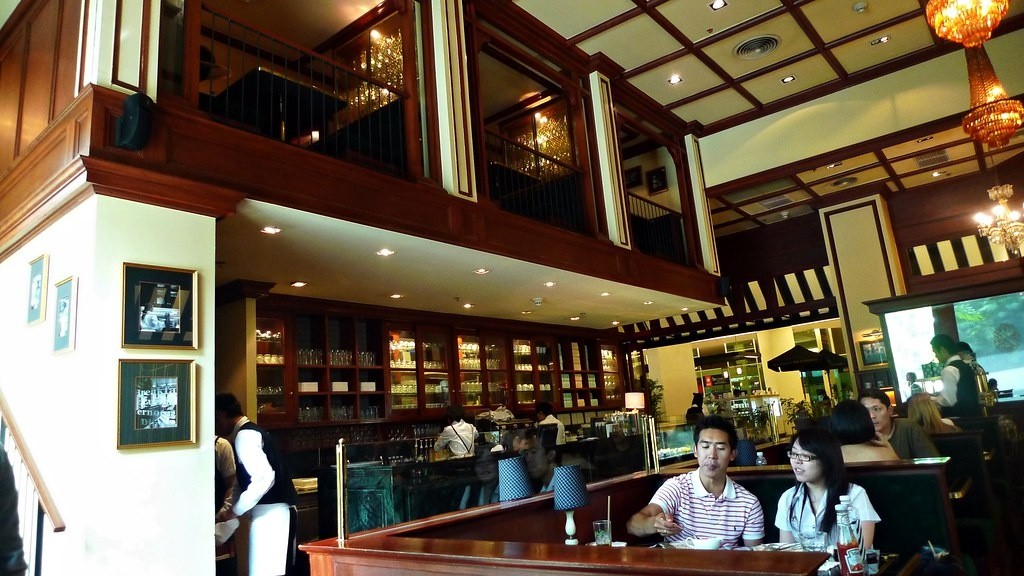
[564,401,573,408]
[598,410,612,417]
[578,399,585,407]
[361,381,376,392]
[591,399,598,406]
[294,476,318,491]
[585,411,596,422]
[562,381,571,388]
[563,392,572,401]
[332,381,348,392]
[572,342,579,350]
[573,357,581,363]
[576,381,583,388]
[573,350,579,356]
[300,382,320,392]
[558,414,570,425]
[562,374,569,381]
[572,412,584,424]
[575,374,582,381]
[589,380,597,387]
[573,364,581,370]
[588,374,596,381]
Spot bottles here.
[256,330,284,341]
[257,354,285,365]
[835,505,864,576]
[296,348,377,366]
[611,412,636,435]
[332,405,379,419]
[756,452,767,465]
[388,455,404,464]
[299,407,323,422]
[839,495,867,575]
[390,339,501,391]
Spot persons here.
[459,421,597,511]
[626,414,765,551]
[434,407,480,459]
[215,434,237,526]
[684,392,710,426]
[532,401,567,445]
[490,427,533,453]
[773,424,881,552]
[139,303,162,334]
[831,333,1000,462]
[215,391,299,576]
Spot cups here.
[258,386,282,395]
[685,537,723,550]
[593,520,612,547]
[517,383,551,390]
[514,345,548,370]
[799,531,828,552]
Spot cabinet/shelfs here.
[638,211,687,266]
[500,173,587,235]
[255,292,625,432]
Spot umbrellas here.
[768,344,831,399]
[799,348,848,399]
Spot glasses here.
[957,352,970,357]
[787,450,820,462]
[472,458,496,468]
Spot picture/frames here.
[645,166,669,197]
[27,252,50,325]
[122,261,201,351]
[626,164,643,187]
[52,274,79,353]
[114,358,198,451]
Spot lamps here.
[498,455,528,502]
[729,437,757,467]
[625,391,645,412]
[348,21,402,110]
[507,110,574,179]
[554,465,592,546]
[962,44,1024,149]
[927,0,1009,48]
[972,148,1024,259]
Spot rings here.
[664,530,667,534]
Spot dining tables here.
[842,551,923,576]
[947,461,978,500]
[983,444,995,462]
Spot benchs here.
[942,456,981,576]
[953,418,1021,552]
[645,469,949,572]
[935,432,1016,576]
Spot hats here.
[956,341,973,353]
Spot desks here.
[213,65,349,156]
[490,161,543,205]
